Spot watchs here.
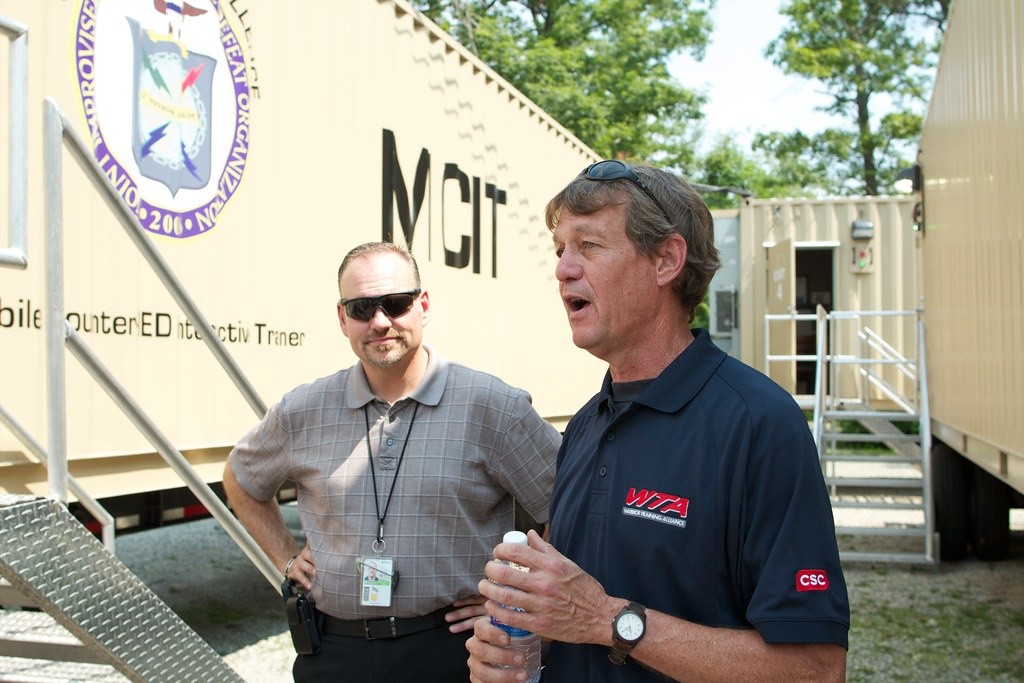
[608,599,649,667]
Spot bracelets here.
[285,553,304,587]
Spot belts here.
[314,604,474,640]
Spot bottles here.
[489,531,541,683]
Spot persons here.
[463,163,849,683]
[363,563,380,583]
[220,241,565,683]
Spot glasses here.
[342,288,421,322]
[578,160,672,224]
